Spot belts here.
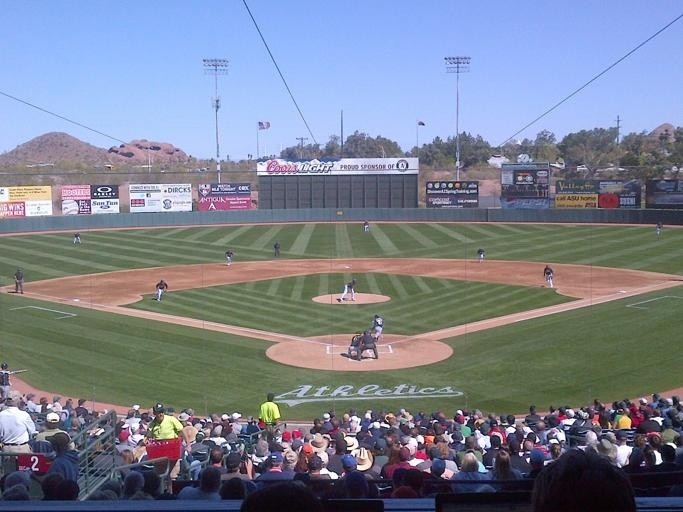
[3,442,27,446]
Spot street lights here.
[199,55,231,184]
[443,54,472,183]
[414,117,425,159]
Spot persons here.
[355,330,379,359]
[364,220,369,232]
[655,221,663,239]
[544,264,554,287]
[13,268,24,294]
[225,249,235,266]
[273,242,280,256]
[73,233,81,244]
[340,278,356,301]
[371,315,384,340]
[0,364,250,500]
[239,393,683,511]
[347,331,363,360]
[155,280,168,301]
[477,248,485,262]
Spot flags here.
[417,120,425,126]
[259,122,271,129]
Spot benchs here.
[562,424,639,448]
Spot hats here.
[5,390,22,402]
[268,399,673,474]
[221,412,242,420]
[178,412,190,422]
[153,403,165,412]
[46,412,60,422]
[184,426,198,444]
[45,433,69,446]
[226,452,241,468]
